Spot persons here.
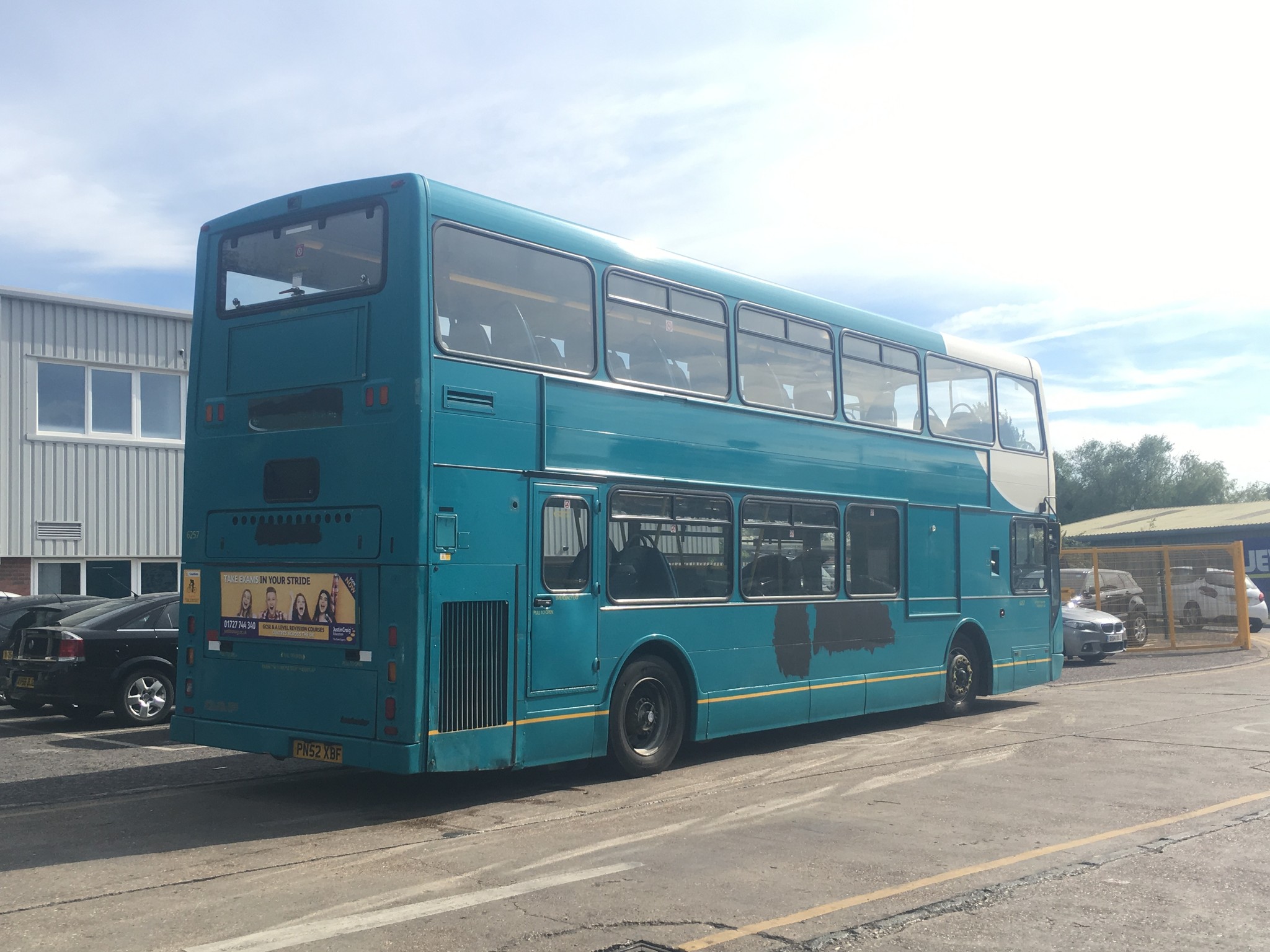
[259,587,287,620]
[288,590,312,621]
[236,589,257,619]
[312,590,337,622]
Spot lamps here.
[180,348,185,363]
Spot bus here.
[170,172,1064,778]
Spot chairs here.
[445,303,1019,448]
[562,534,679,599]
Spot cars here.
[0,591,119,709]
[1017,570,1148,648]
[7,593,180,728]
[1144,566,1268,633]
[1061,607,1128,663]
